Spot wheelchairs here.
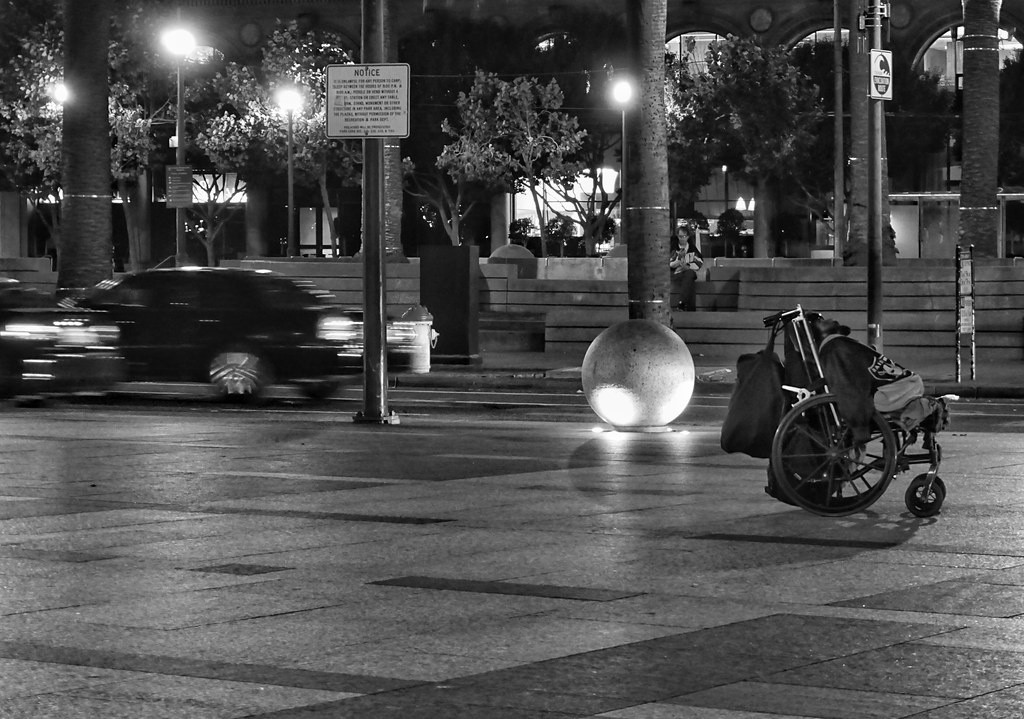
[761,303,960,516]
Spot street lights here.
[612,77,629,258]
[272,86,307,257]
[158,5,202,263]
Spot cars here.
[0,265,437,404]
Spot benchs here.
[217,257,1024,368]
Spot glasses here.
[678,235,687,239]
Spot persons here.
[670,227,705,311]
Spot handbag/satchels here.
[721,323,782,457]
